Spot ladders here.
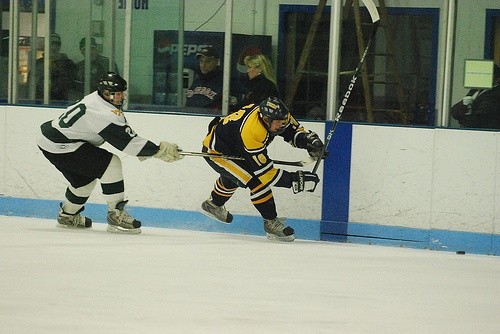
[284,0,412,128]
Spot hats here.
[195,46,220,60]
[50,33,63,43]
[79,36,97,50]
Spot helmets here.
[256,96,290,137]
[97,71,128,108]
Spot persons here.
[26,34,74,101]
[35,71,185,235]
[185,47,223,109]
[452,87,499,130]
[206,50,280,116]
[69,37,119,102]
[200,96,330,242]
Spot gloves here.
[154,141,185,164]
[304,130,330,159]
[291,170,320,194]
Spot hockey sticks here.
[177,149,318,167]
[310,1,381,176]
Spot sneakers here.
[106,200,143,234]
[55,202,92,230]
[262,218,296,242]
[201,199,234,225]
